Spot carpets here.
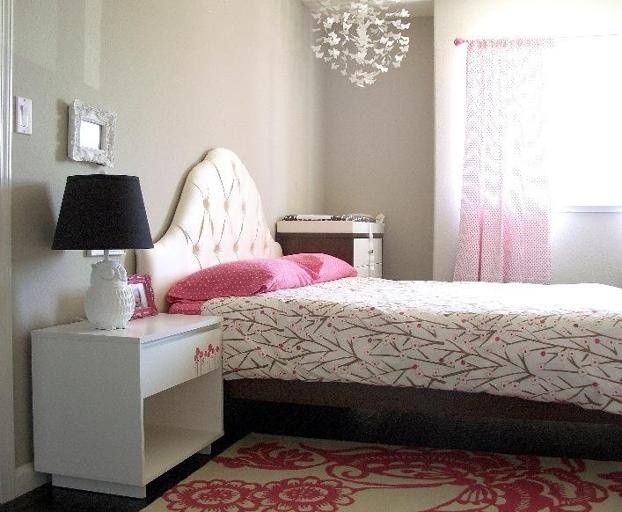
[141,431,621,512]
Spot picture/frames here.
[68,99,119,166]
[125,275,159,321]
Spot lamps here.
[309,1,410,89]
[51,174,155,330]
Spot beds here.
[136,147,620,462]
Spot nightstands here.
[30,311,225,498]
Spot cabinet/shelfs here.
[275,222,383,278]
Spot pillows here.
[165,253,358,304]
